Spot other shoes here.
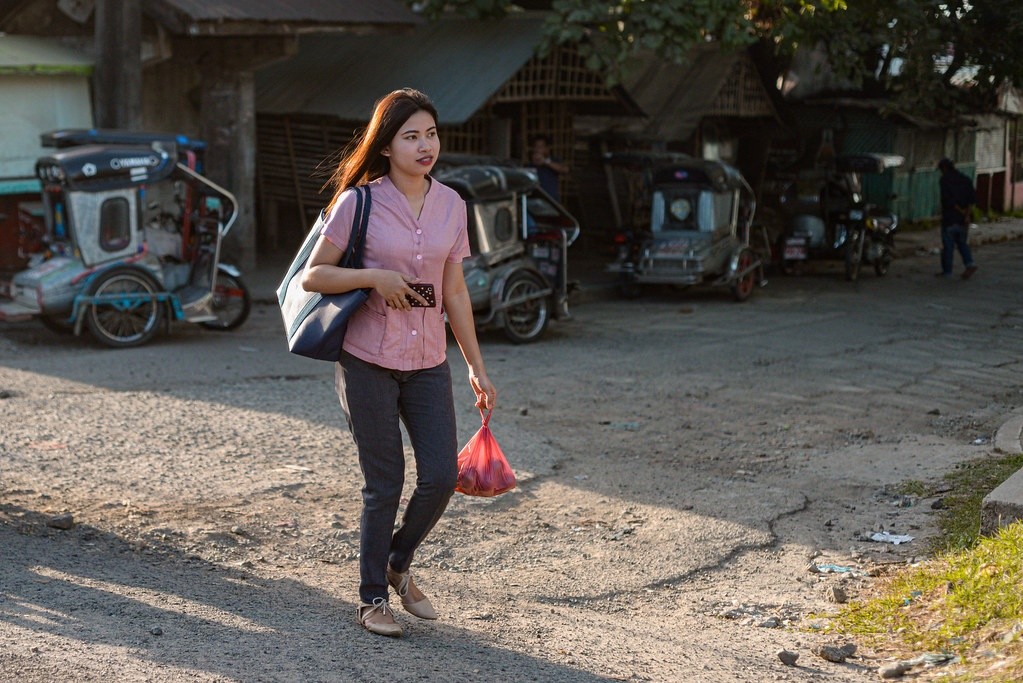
[356,597,403,636]
[933,272,951,278]
[386,567,438,620]
[961,266,977,279]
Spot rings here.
[400,299,407,302]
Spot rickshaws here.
[768,151,908,282]
[430,151,581,345]
[0,126,251,351]
[603,158,777,307]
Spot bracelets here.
[545,159,551,164]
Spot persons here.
[300,86,495,637]
[934,158,977,280]
[527,136,568,200]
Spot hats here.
[939,159,954,171]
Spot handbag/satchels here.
[454,405,516,498]
[276,185,374,362]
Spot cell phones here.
[386,283,437,308]
